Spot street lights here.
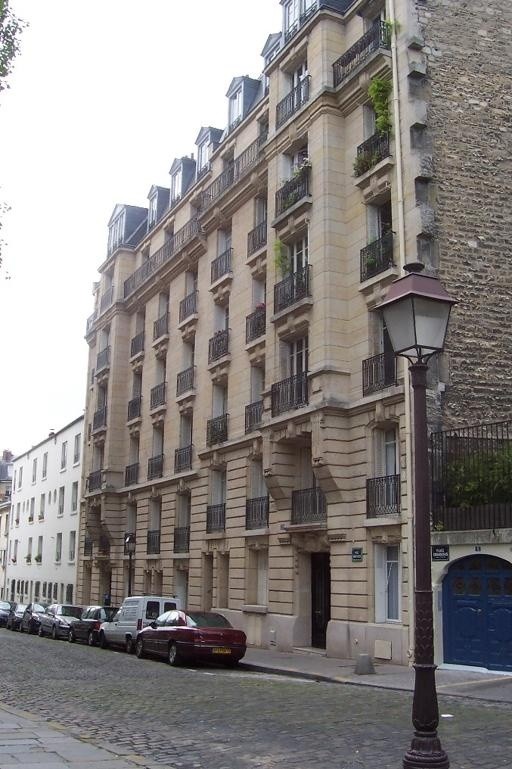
[371,261,461,767]
[124,533,136,595]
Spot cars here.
[68,605,121,645]
[133,607,248,668]
[38,603,85,640]
[19,602,51,634]
[0,600,17,628]
[6,603,30,631]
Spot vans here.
[98,596,182,653]
